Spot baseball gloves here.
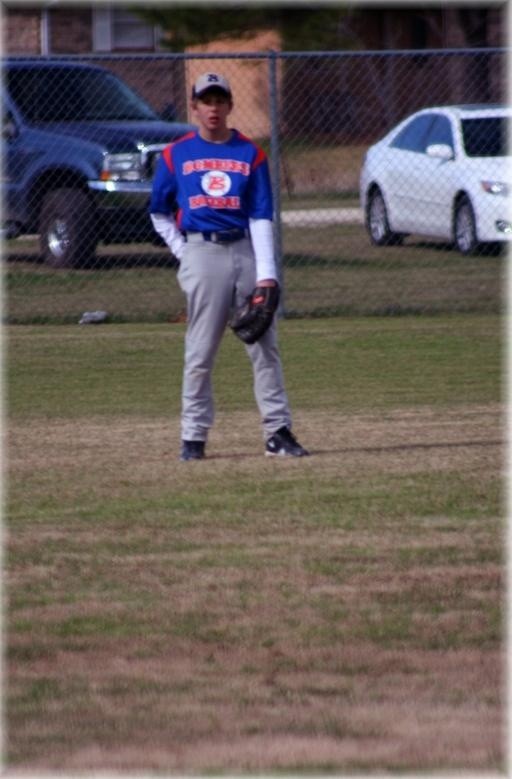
[230,287,280,344]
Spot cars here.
[356,102,511,252]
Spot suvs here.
[0,54,198,265]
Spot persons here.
[146,73,308,459]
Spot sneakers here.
[181,441,204,460]
[266,426,309,458]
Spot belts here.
[186,231,244,242]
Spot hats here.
[191,72,231,97]
